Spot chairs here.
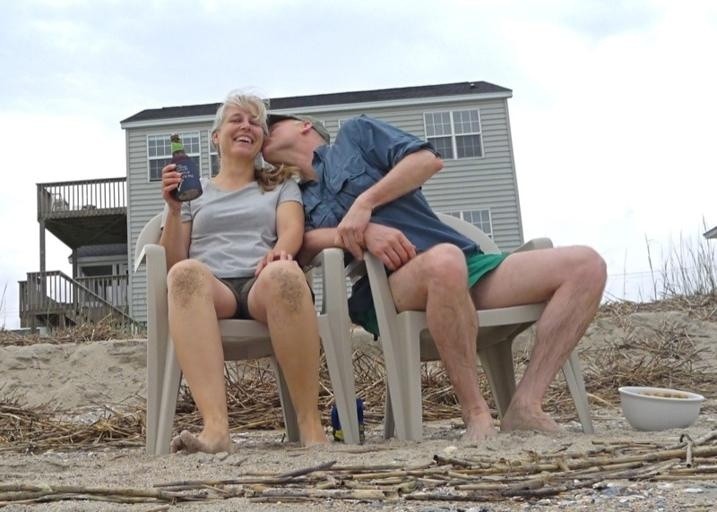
[130,212,361,457]
[344,212,594,440]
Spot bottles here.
[170,133,202,203]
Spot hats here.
[266,112,330,144]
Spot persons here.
[262,115,606,440]
[156,95,328,451]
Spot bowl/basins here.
[615,386,704,430]
